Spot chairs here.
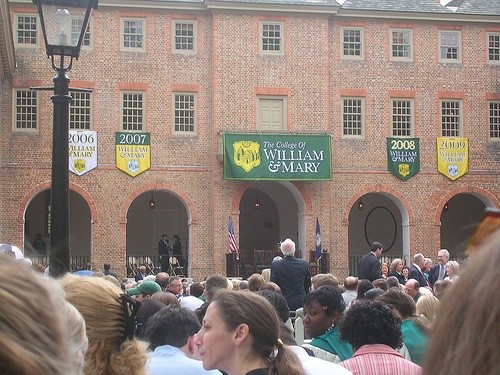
[128,256,155,278]
[169,257,185,278]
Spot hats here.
[126,280,162,295]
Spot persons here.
[0,209,500,375]
[168,235,184,267]
[158,234,170,273]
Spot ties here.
[439,266,444,280]
[422,271,432,287]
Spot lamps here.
[254,182,261,208]
[443,204,448,211]
[358,199,364,210]
[149,191,155,209]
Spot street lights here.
[32,0,100,279]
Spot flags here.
[315,219,323,261]
[227,219,239,259]
